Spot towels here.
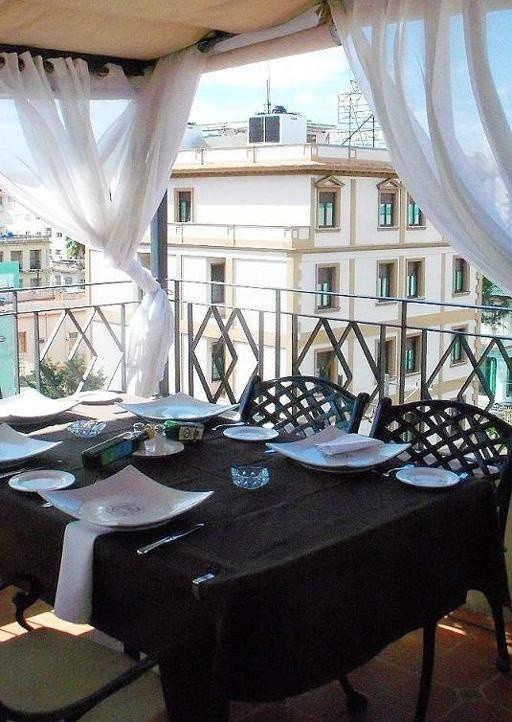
[53,521,114,625]
[315,432,383,456]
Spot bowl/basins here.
[66,420,107,439]
[231,466,270,490]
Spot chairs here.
[0,571,182,722]
[364,398,512,722]
[238,375,368,434]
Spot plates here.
[223,425,279,441]
[37,463,216,527]
[72,391,121,405]
[130,437,184,458]
[0,388,82,425]
[7,469,76,494]
[75,392,120,406]
[115,391,241,426]
[0,421,65,468]
[395,465,461,489]
[265,425,412,475]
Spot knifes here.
[0,465,44,480]
[255,449,279,455]
[264,448,276,454]
[136,515,206,557]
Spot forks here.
[207,421,245,434]
[376,462,416,480]
[40,479,100,510]
[378,466,406,480]
[210,421,245,431]
